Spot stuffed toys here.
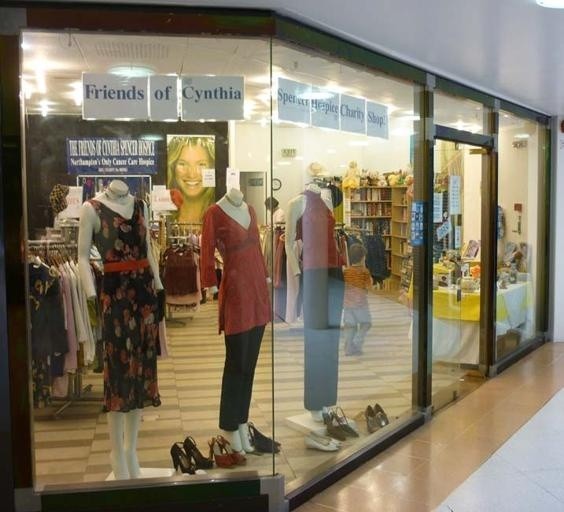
[343,160,414,196]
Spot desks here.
[430,279,528,364]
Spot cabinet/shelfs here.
[342,187,415,290]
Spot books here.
[350,186,413,291]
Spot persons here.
[265,196,285,230]
[191,228,198,246]
[343,243,372,357]
[74,178,165,482]
[196,230,202,247]
[166,136,217,226]
[283,183,349,423]
[203,187,273,461]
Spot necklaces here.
[104,191,132,206]
[225,194,243,207]
[108,186,131,197]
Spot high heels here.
[207,436,235,467]
[247,420,282,454]
[217,434,247,465]
[183,436,214,470]
[169,441,196,475]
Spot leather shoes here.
[303,430,344,453]
[364,402,390,434]
[322,406,360,440]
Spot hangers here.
[266,222,286,232]
[25,244,78,267]
[174,221,203,253]
